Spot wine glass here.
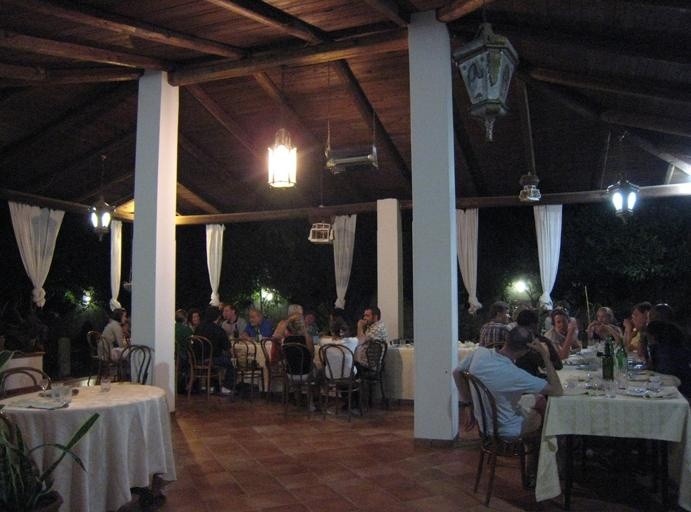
[40,379,48,397]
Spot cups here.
[400,340,406,348]
[101,377,111,392]
[60,383,74,405]
[51,382,65,405]
[392,339,398,348]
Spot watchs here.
[461,402,475,409]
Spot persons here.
[221,305,246,336]
[479,301,512,348]
[638,303,691,406]
[96,308,129,380]
[173,308,194,394]
[272,305,302,340]
[188,312,199,332]
[451,325,568,506]
[510,302,529,320]
[326,309,350,338]
[507,306,562,371]
[586,308,623,346]
[303,313,319,337]
[282,316,318,414]
[623,302,649,352]
[239,310,273,397]
[543,310,581,357]
[341,308,388,412]
[195,308,235,397]
[544,302,578,345]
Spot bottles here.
[254,324,262,343]
[607,334,614,359]
[232,323,239,344]
[601,340,614,382]
[616,337,628,373]
[312,328,318,345]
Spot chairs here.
[459,368,540,508]
[260,335,283,401]
[0,366,50,403]
[84,326,111,382]
[226,338,264,404]
[315,343,358,418]
[184,334,219,402]
[347,338,390,411]
[111,346,153,386]
[277,342,317,418]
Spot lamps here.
[451,5,522,144]
[86,153,116,241]
[264,60,300,191]
[518,84,541,207]
[605,128,643,220]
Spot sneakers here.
[220,388,234,395]
